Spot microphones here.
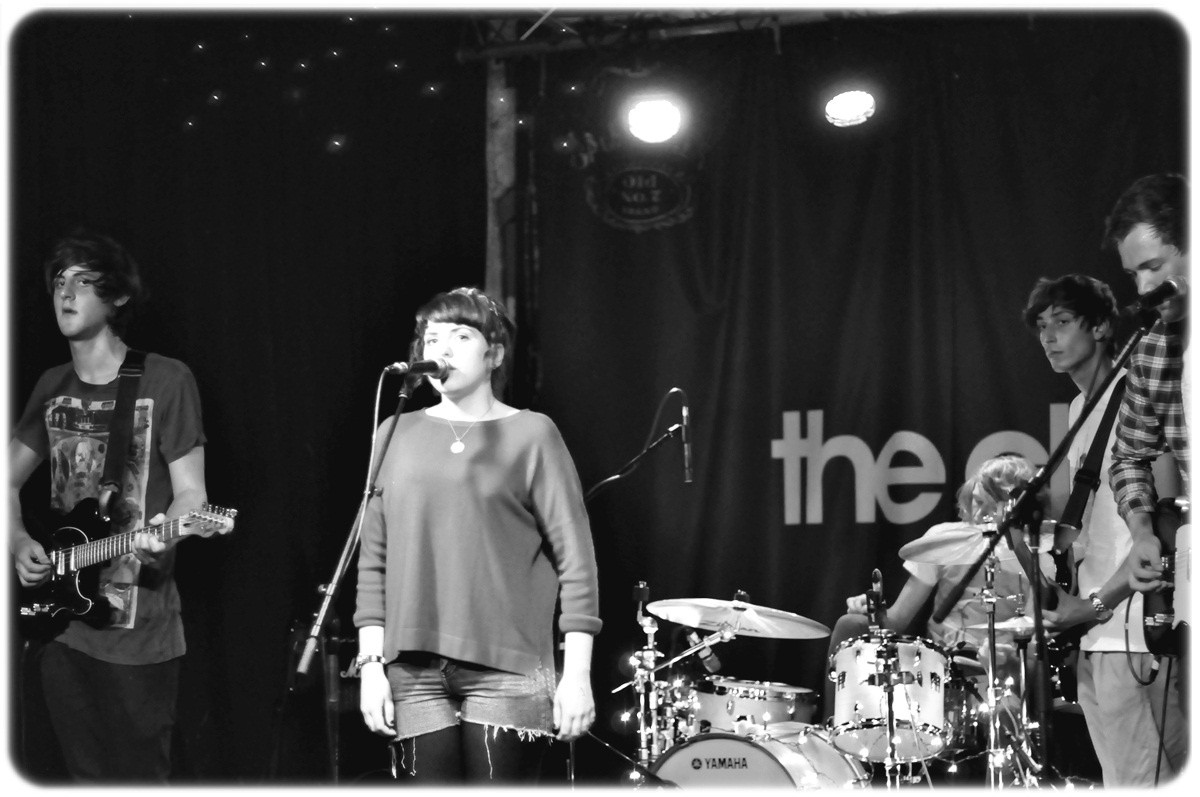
[685,628,721,672]
[1123,275,1190,320]
[384,356,450,379]
[681,406,694,482]
[871,569,888,628]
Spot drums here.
[828,634,952,764]
[643,722,871,788]
[685,676,816,733]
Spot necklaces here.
[441,397,496,453]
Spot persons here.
[826,431,1052,723]
[12,226,208,786]
[1101,172,1188,771]
[353,287,602,784]
[1024,273,1175,785]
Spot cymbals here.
[645,597,832,640]
[965,618,1052,637]
[898,519,1057,565]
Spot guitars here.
[1143,497,1191,658]
[13,498,238,647]
[980,475,1081,659]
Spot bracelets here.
[356,653,386,668]
[1090,592,1105,615]
